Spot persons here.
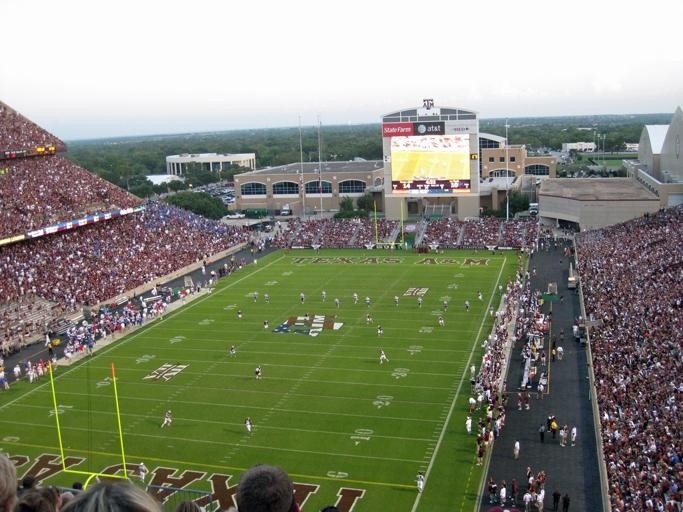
[0,101,683,512]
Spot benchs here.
[1,291,85,361]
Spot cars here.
[280,208,291,216]
[226,211,245,219]
[529,207,538,216]
[197,182,235,206]
[556,155,576,178]
[543,151,550,154]
[556,148,561,152]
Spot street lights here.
[593,131,596,160]
[188,184,192,192]
[503,119,511,221]
[597,134,600,163]
[602,134,606,161]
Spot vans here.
[529,203,538,208]
